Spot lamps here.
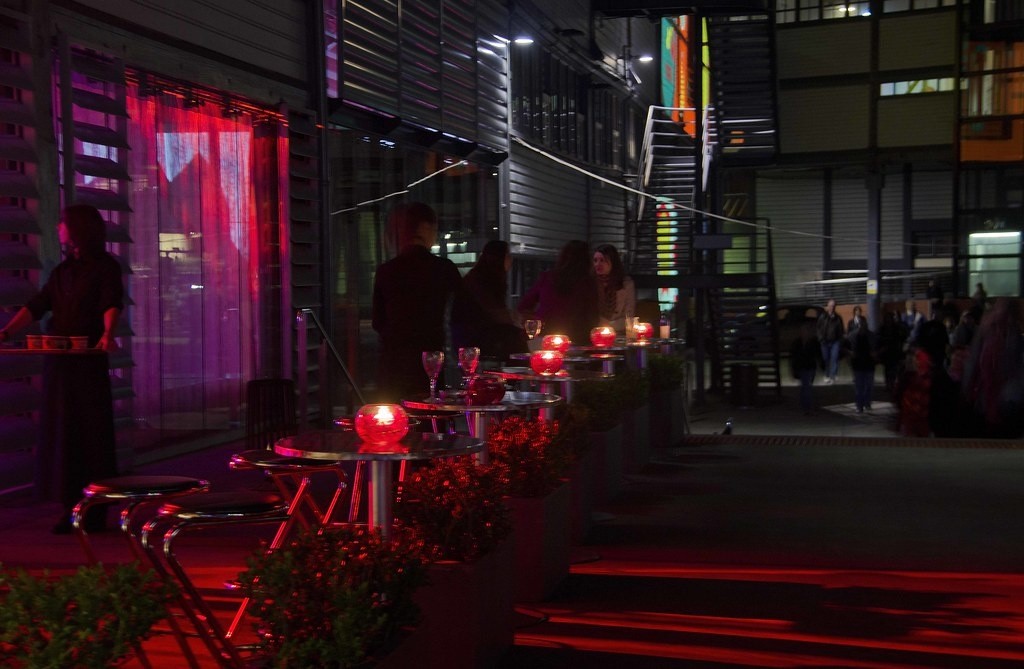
[634,52,654,63]
[554,26,586,38]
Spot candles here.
[590,326,617,348]
[353,403,411,444]
[530,351,562,378]
[542,334,571,358]
[637,322,654,341]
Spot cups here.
[659,318,671,339]
[625,317,636,339]
[525,320,542,339]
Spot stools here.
[71,380,523,669]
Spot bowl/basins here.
[26,334,89,350]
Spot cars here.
[706,303,826,360]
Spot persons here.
[454,239,543,386]
[789,320,821,409]
[881,298,975,407]
[0,200,130,536]
[546,240,602,357]
[926,280,947,315]
[371,201,486,416]
[581,243,646,381]
[844,307,872,357]
[817,298,843,382]
[844,315,879,413]
[971,280,986,305]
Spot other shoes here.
[856,406,873,413]
[53,507,109,534]
[824,376,833,384]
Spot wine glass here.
[458,347,480,387]
[422,350,445,403]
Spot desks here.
[275,337,685,534]
